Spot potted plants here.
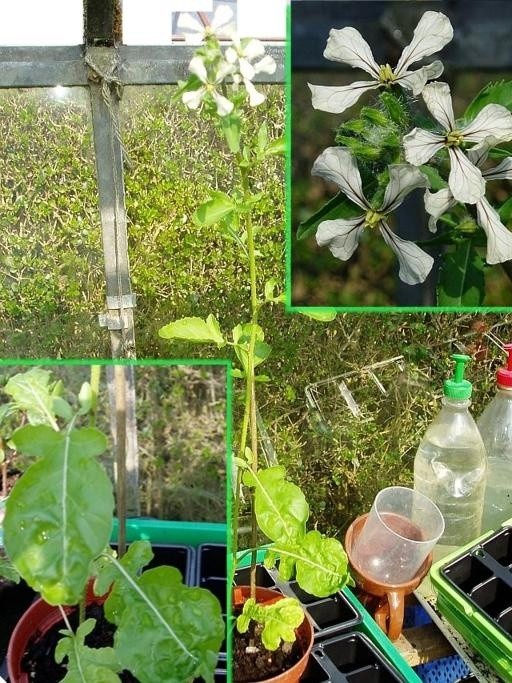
[1,365,226,681]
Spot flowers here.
[159,4,354,652]
[296,8,512,308]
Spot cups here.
[343,487,446,645]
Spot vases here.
[231,584,313,682]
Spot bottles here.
[411,345,512,599]
